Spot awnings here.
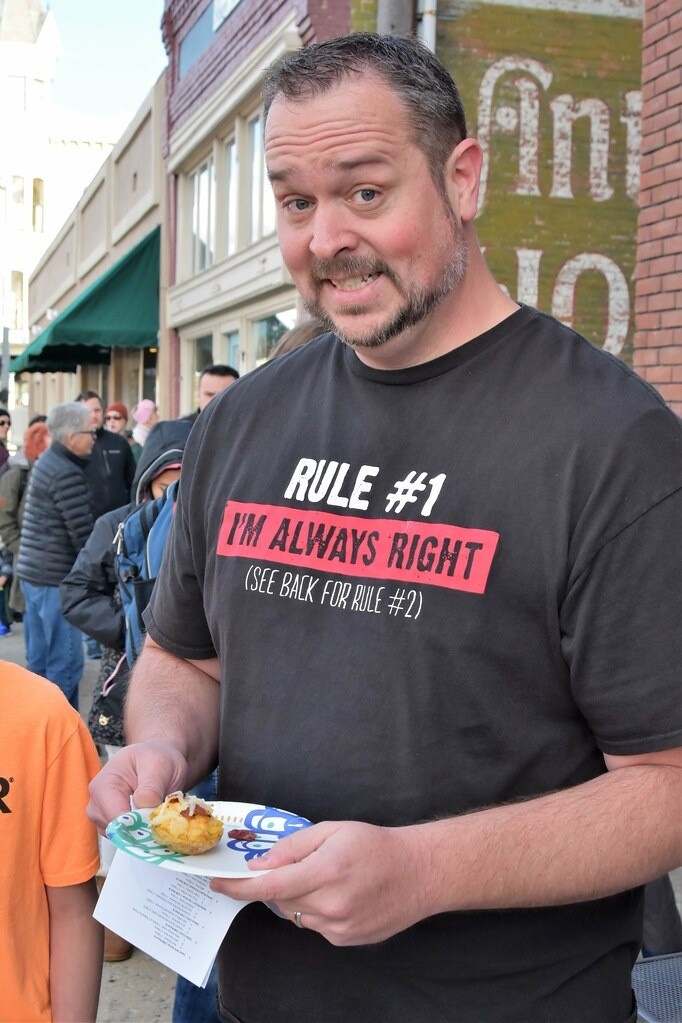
[8,223,160,373]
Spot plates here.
[105,802,314,879]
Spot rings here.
[294,911,304,928]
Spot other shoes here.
[103,926,133,961]
[13,612,23,622]
[88,647,102,658]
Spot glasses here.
[105,415,124,420]
[0,420,10,425]
[73,427,97,441]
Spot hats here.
[148,459,181,480]
[24,422,51,460]
[132,399,154,424]
[105,402,128,422]
[0,408,10,418]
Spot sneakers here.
[0,623,13,638]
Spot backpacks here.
[112,477,181,670]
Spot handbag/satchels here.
[87,641,130,746]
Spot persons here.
[0,367,240,964]
[84,33,682,1023]
[0,657,105,1022]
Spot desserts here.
[149,791,226,856]
